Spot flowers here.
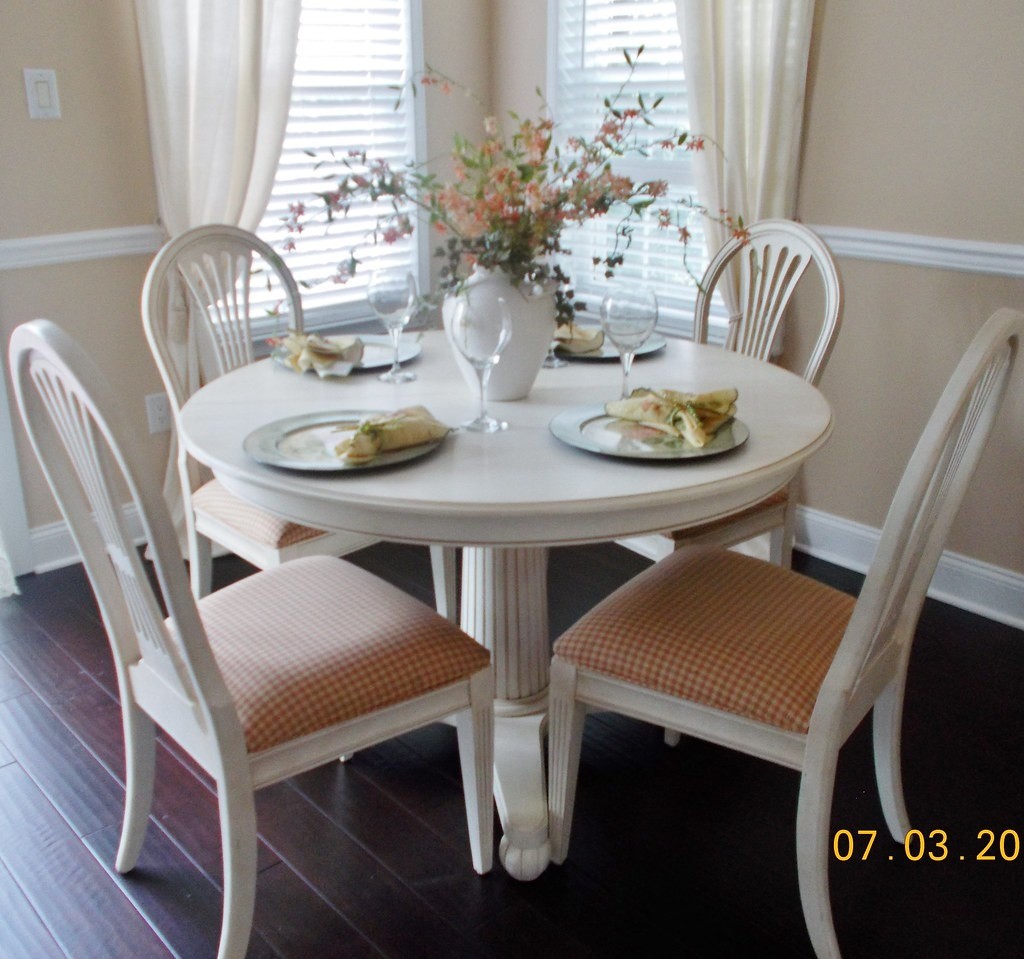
[279,44,767,345]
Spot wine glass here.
[369,270,417,383]
[450,298,514,433]
[600,284,660,400]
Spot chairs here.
[656,218,844,569]
[142,221,381,599]
[548,306,1024,959]
[9,317,496,959]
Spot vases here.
[442,263,557,401]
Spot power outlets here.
[145,392,172,434]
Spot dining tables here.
[178,329,835,880]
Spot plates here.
[271,334,421,369]
[242,410,441,470]
[549,399,749,459]
[557,324,665,359]
